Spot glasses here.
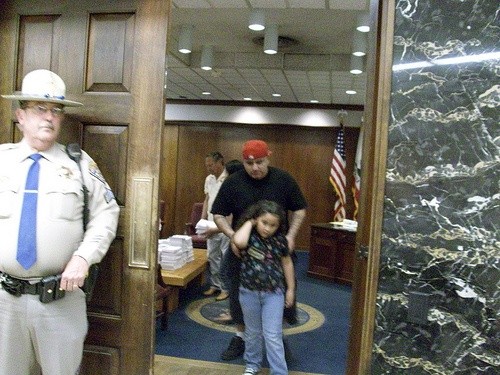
[24,104,66,118]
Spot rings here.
[73,285,78,287]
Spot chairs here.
[184,203,208,249]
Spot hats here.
[242,139,273,160]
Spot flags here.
[351,124,365,220]
[329,123,346,226]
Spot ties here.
[17,154,43,270]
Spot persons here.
[201,152,229,301]
[232,200,294,375]
[0,69,120,375]
[225,160,244,175]
[210,140,308,362]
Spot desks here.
[160,248,211,313]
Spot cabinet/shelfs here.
[306,222,358,286]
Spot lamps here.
[201,46,216,70]
[356,11,371,33]
[349,53,364,74]
[248,9,265,31]
[352,32,367,56]
[264,22,279,54]
[178,23,193,54]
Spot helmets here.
[1,69,84,107]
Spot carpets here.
[154,252,352,375]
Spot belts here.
[20,280,43,295]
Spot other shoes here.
[222,335,246,361]
[216,289,228,300]
[204,288,216,296]
[242,367,261,375]
[284,313,296,326]
